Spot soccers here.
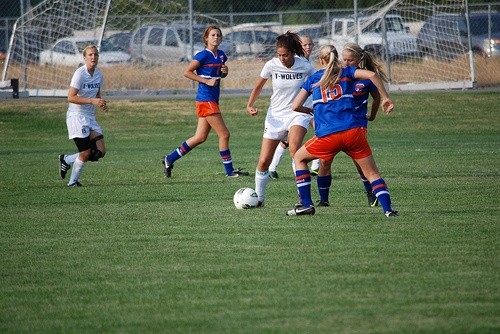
[233,187,259,210]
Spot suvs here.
[39,11,500,69]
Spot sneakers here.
[160,155,173,178]
[258,200,264,207]
[226,167,250,178]
[385,210,398,217]
[59,153,70,180]
[269,171,279,181]
[365,191,378,207]
[316,200,329,207]
[285,204,315,216]
[295,202,303,206]
[67,180,83,187]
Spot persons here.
[315,42,378,207]
[160,27,249,178]
[268,33,319,180]
[286,45,399,217]
[58,46,108,187]
[247,30,317,207]
